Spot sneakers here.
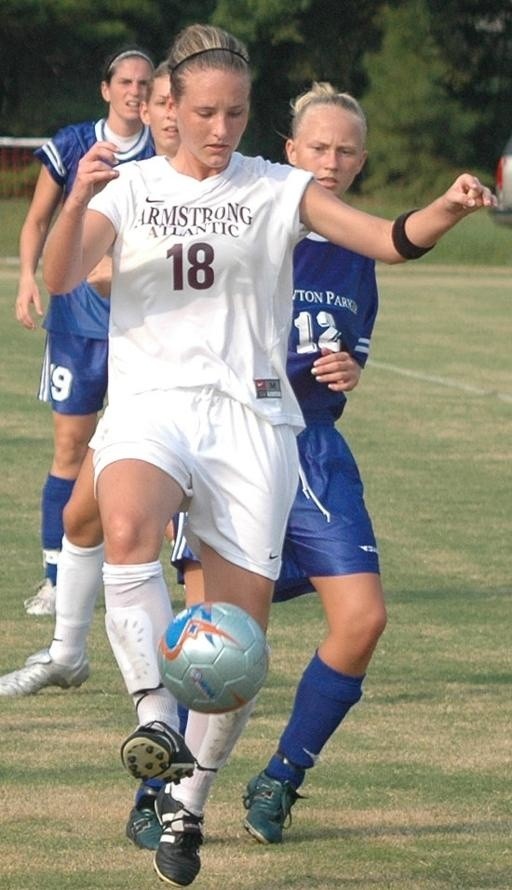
[0,648,92,699]
[239,768,299,846]
[119,716,195,789]
[152,784,206,888]
[22,577,56,616]
[125,804,163,851]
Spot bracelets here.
[392,210,439,261]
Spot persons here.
[14,42,155,615]
[42,16,506,888]
[0,58,181,699]
[120,77,391,850]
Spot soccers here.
[157,600,269,713]
